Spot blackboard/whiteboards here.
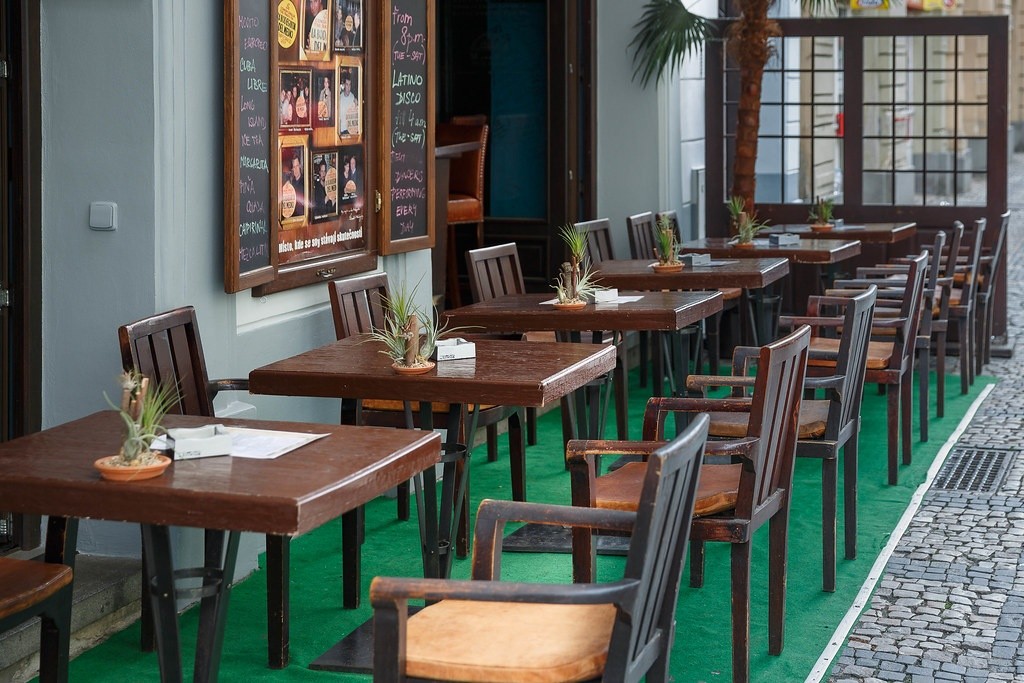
[222,0,278,294]
[379,0,435,256]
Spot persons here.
[276,0,363,224]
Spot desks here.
[762,221,916,341]
[438,291,723,557]
[249,332,616,676]
[0,410,441,683]
[431,141,480,333]
[579,259,790,473]
[674,237,861,348]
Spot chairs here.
[430,124,499,309]
[450,115,487,124]
[0,208,1012,683]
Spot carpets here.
[29,355,1001,683]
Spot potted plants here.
[808,196,835,233]
[650,215,685,273]
[727,195,771,250]
[93,369,186,482]
[355,270,435,376]
[550,219,604,310]
